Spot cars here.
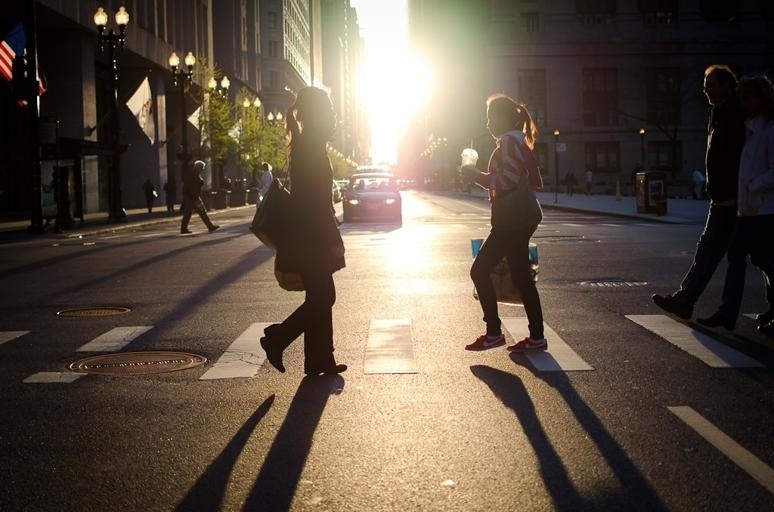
[332,172,401,223]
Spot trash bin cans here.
[636,171,667,214]
[230,178,247,208]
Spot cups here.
[458,148,479,185]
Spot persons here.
[260,87,349,375]
[456,94,550,351]
[142,178,156,213]
[563,167,575,196]
[251,163,263,187]
[164,175,177,212]
[734,74,773,332]
[253,162,275,198]
[652,64,747,331]
[178,160,220,234]
[584,168,594,196]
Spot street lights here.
[639,128,645,170]
[553,129,560,205]
[93,6,130,224]
[168,50,197,179]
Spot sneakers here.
[305,362,347,377]
[259,336,286,374]
[648,293,696,321]
[462,331,506,350]
[507,336,548,353]
[755,307,774,333]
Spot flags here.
[0,24,48,107]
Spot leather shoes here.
[695,306,738,330]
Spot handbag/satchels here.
[467,234,540,308]
[249,177,294,251]
[490,179,546,242]
